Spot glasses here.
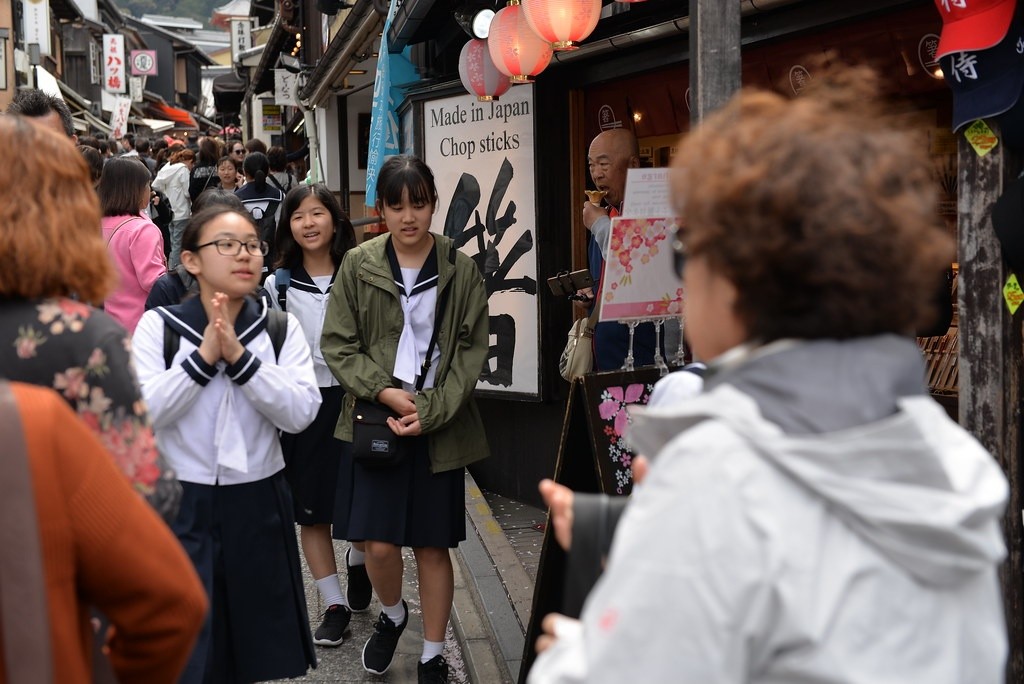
[197,238,269,256]
[234,149,246,155]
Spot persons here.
[571,129,667,373]
[0,88,490,684]
[522,87,1008,684]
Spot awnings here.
[212,74,246,115]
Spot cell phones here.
[547,269,594,297]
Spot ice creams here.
[585,191,607,203]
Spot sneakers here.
[361,599,409,676]
[313,605,352,646]
[418,654,448,684]
[345,547,372,613]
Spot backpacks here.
[242,201,280,258]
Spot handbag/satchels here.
[559,317,593,384]
[345,399,423,469]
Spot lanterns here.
[521,0,602,50]
[488,6,554,85]
[458,39,512,103]
